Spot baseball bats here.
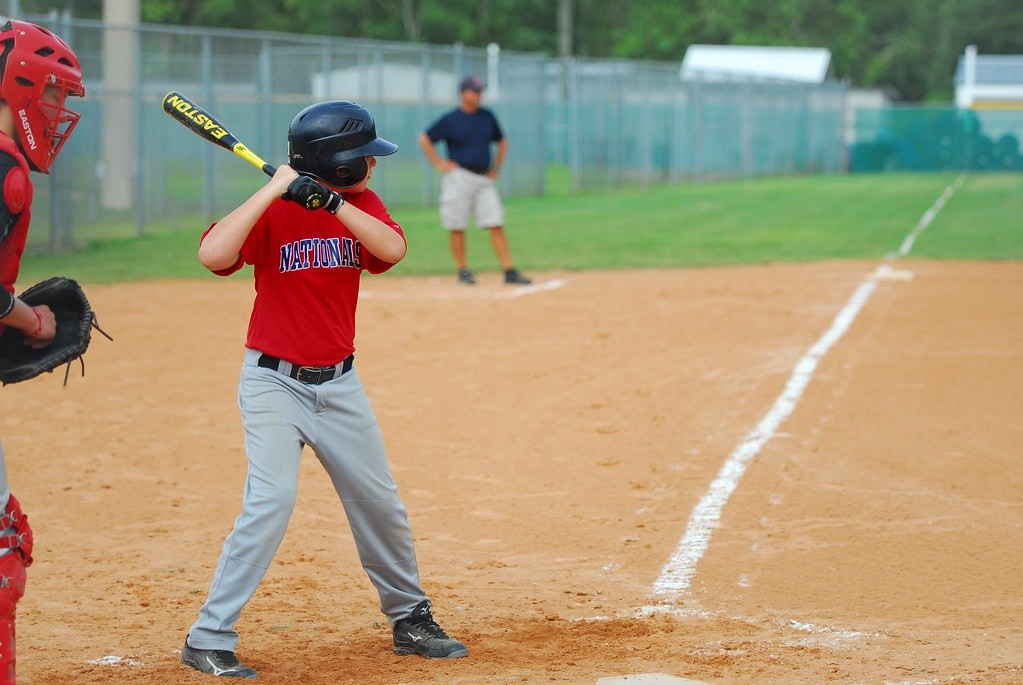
[163,90,321,210]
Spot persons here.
[418,78,532,289]
[175,98,472,684]
[1,16,97,682]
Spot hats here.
[460,76,487,90]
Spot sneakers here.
[458,267,531,284]
[182,633,258,678]
[393,599,470,659]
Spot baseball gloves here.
[0,274,113,386]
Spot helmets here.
[287,100,398,188]
[1,19,85,173]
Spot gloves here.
[282,176,344,214]
